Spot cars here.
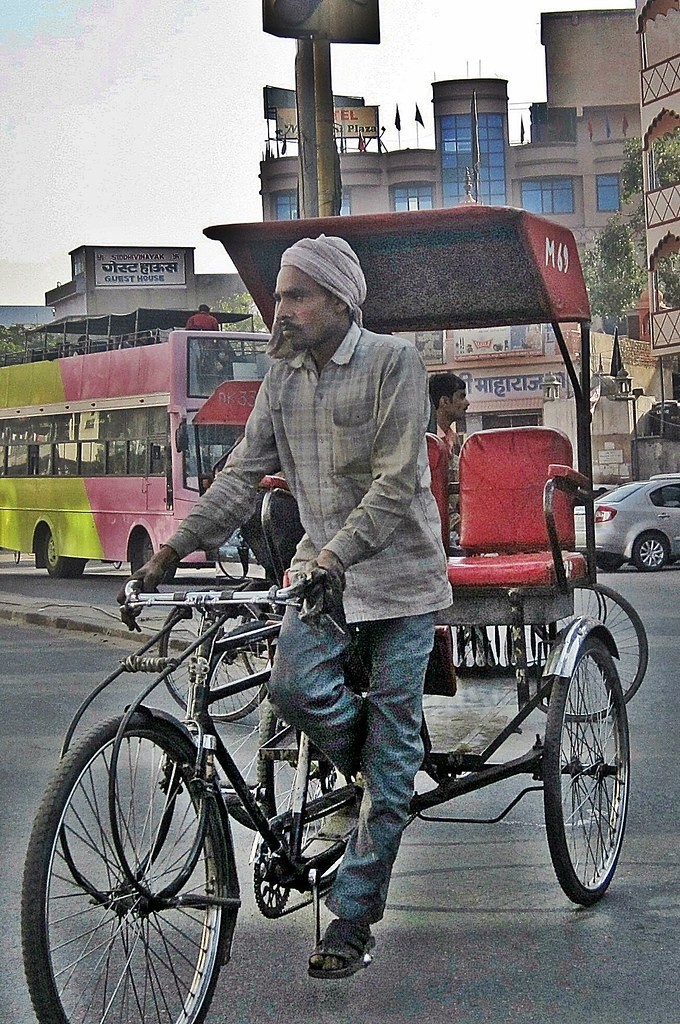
[573,472,680,573]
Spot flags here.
[358,131,365,150]
[415,106,424,127]
[520,119,525,144]
[395,107,401,131]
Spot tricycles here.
[160,380,649,724]
[18,201,632,1022]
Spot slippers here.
[304,919,376,981]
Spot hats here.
[265,233,366,359]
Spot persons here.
[75,304,236,394]
[118,234,455,978]
[429,372,470,533]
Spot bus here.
[0,306,273,586]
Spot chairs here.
[426,432,449,560]
[2,324,176,368]
[444,426,588,585]
